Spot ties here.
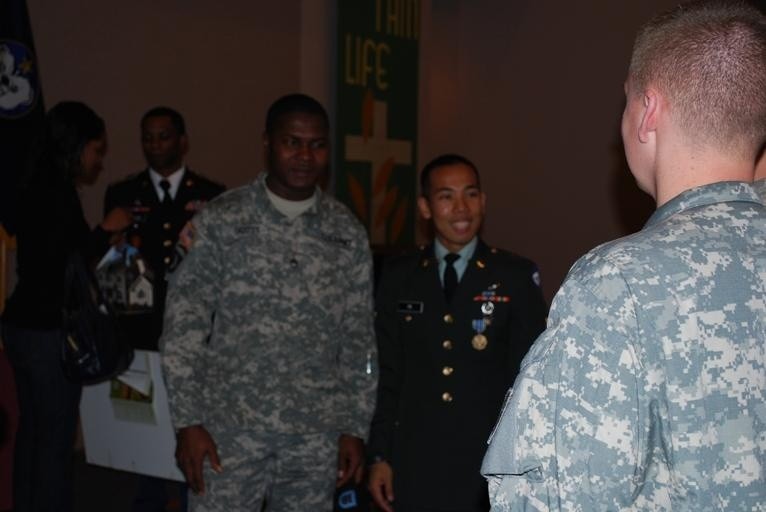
[158,178,175,215]
[441,250,461,301]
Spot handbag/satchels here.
[62,305,134,386]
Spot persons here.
[480,0,766,511]
[1,102,135,511]
[365,153,548,512]
[159,93,381,512]
[100,107,232,511]
